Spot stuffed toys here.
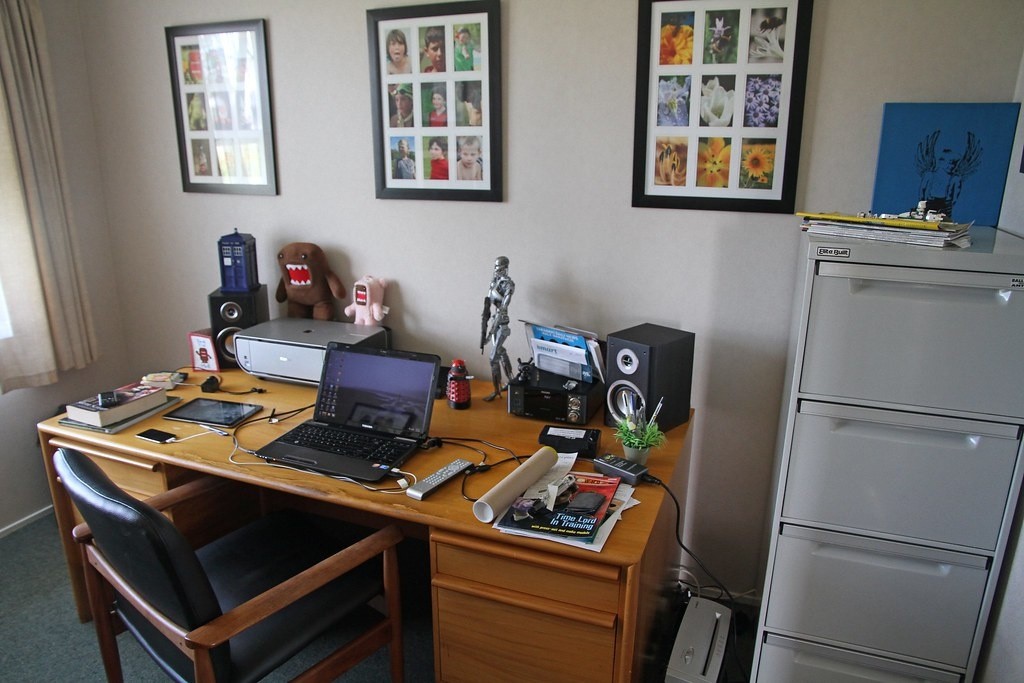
[275,242,346,320]
[344,275,388,324]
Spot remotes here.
[406,458,475,501]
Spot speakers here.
[208,283,270,369]
[602,323,695,437]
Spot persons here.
[385,25,483,180]
[184,44,263,177]
[512,483,597,538]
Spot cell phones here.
[134,428,177,444]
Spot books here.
[529,324,606,383]
[498,473,621,543]
[58,384,181,434]
[140,372,180,390]
[796,212,970,248]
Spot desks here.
[36,365,696,683]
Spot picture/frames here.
[366,0,500,201]
[164,19,278,196]
[629,0,814,213]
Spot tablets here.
[161,397,263,429]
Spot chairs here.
[52,447,405,683]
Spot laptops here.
[256,341,441,483]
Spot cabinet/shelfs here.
[747,218,1024,683]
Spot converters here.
[593,453,649,487]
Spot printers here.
[232,314,386,387]
